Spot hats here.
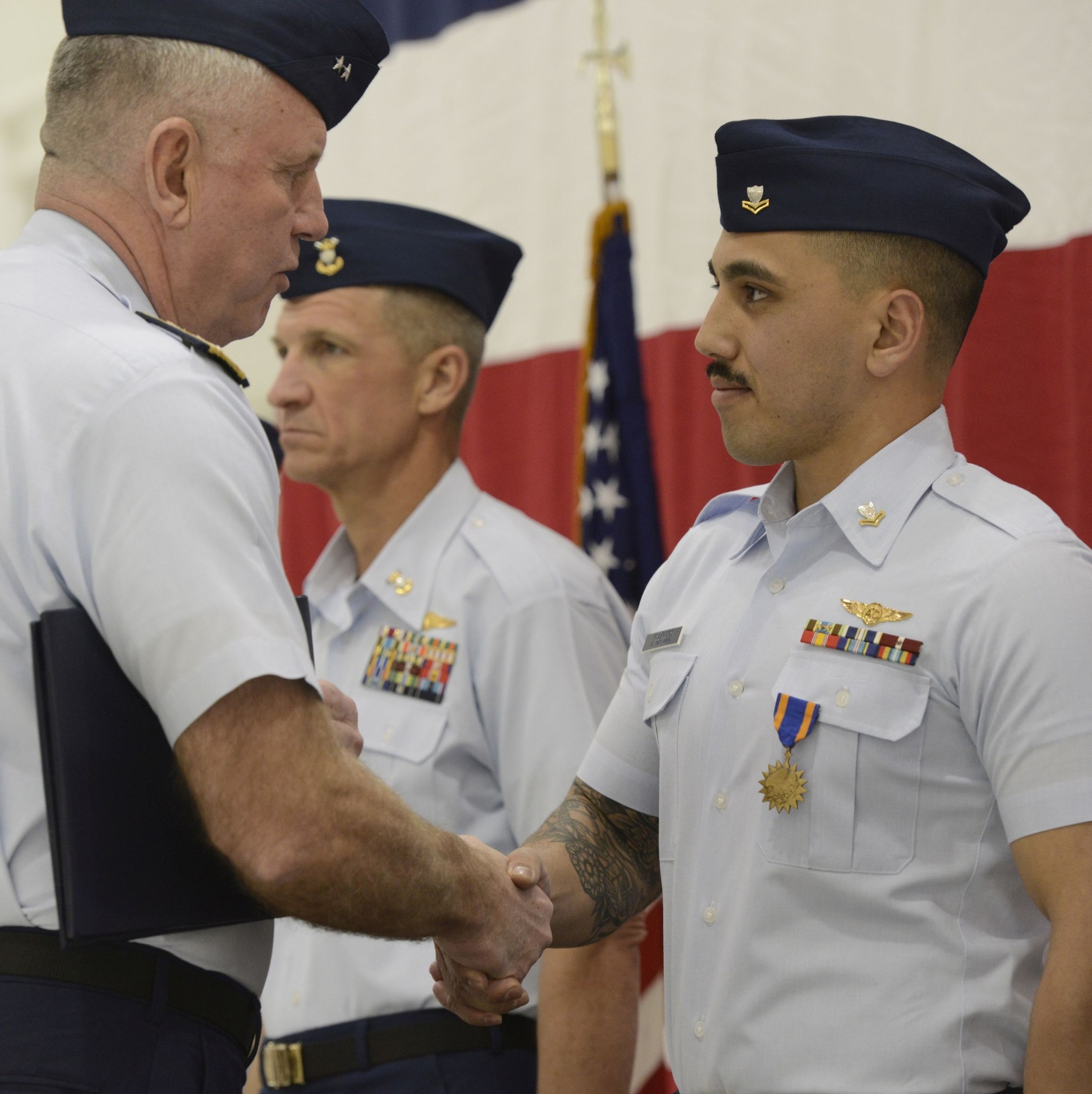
[279,199,523,332]
[715,115,1031,278]
[60,0,390,131]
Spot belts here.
[262,1011,536,1087]
[0,933,259,1053]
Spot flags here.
[573,202,668,613]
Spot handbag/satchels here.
[28,597,314,955]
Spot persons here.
[250,200,633,1094]
[0,0,555,1094]
[433,118,1092,1094]
